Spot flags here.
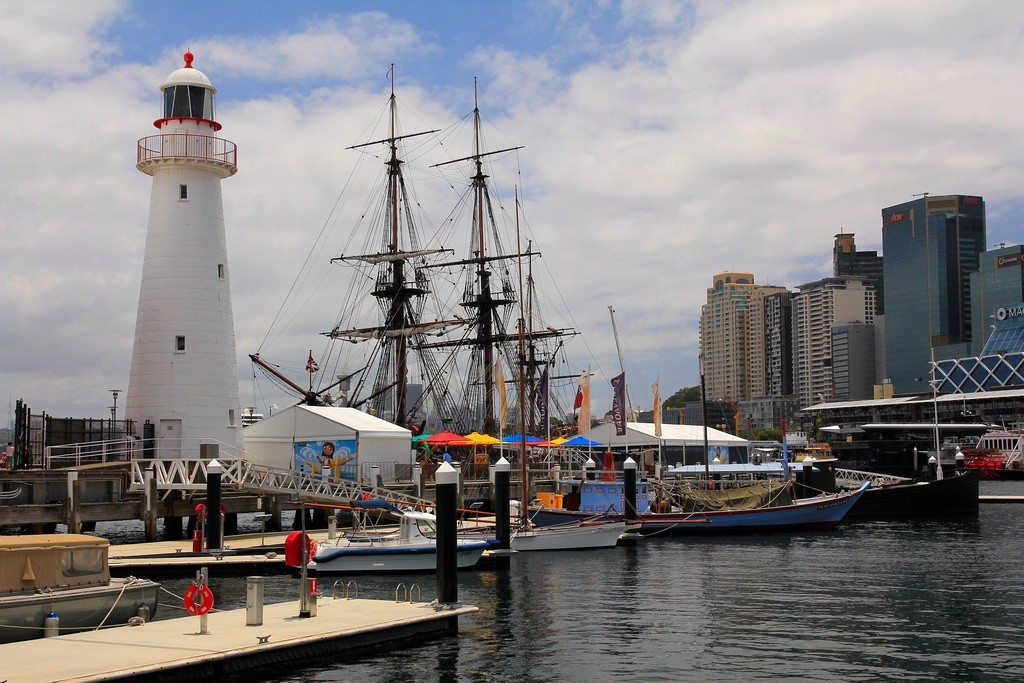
[305,355,319,371]
[611,371,626,436]
[574,368,590,437]
[494,360,507,429]
[651,378,662,437]
[533,365,548,433]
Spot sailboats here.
[249,63,1024,576]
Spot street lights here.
[107,389,123,461]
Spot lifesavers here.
[550,467,554,479]
[195,504,207,522]
[184,584,215,616]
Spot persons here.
[710,447,727,464]
[431,448,451,465]
[6,442,14,469]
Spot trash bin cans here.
[306,578,318,616]
[328,515,337,539]
[247,576,264,625]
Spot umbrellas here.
[418,430,475,451]
[559,435,602,471]
[412,433,433,442]
[466,433,569,479]
